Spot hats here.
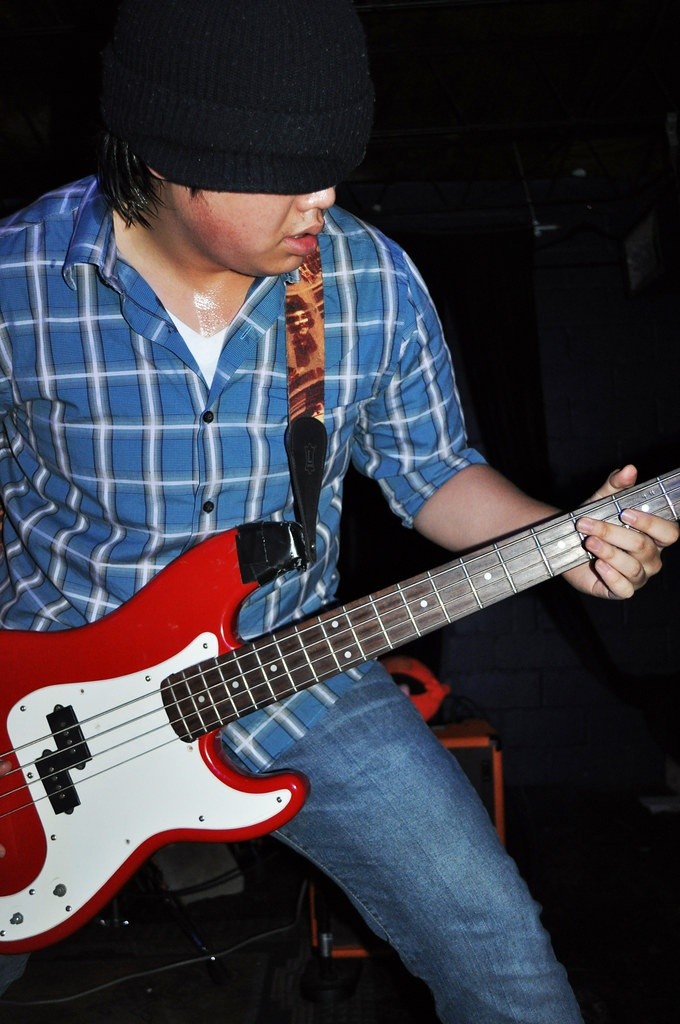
[97,0,374,194]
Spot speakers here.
[308,717,506,957]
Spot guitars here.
[0,473,679,962]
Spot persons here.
[0,0,680,1024]
